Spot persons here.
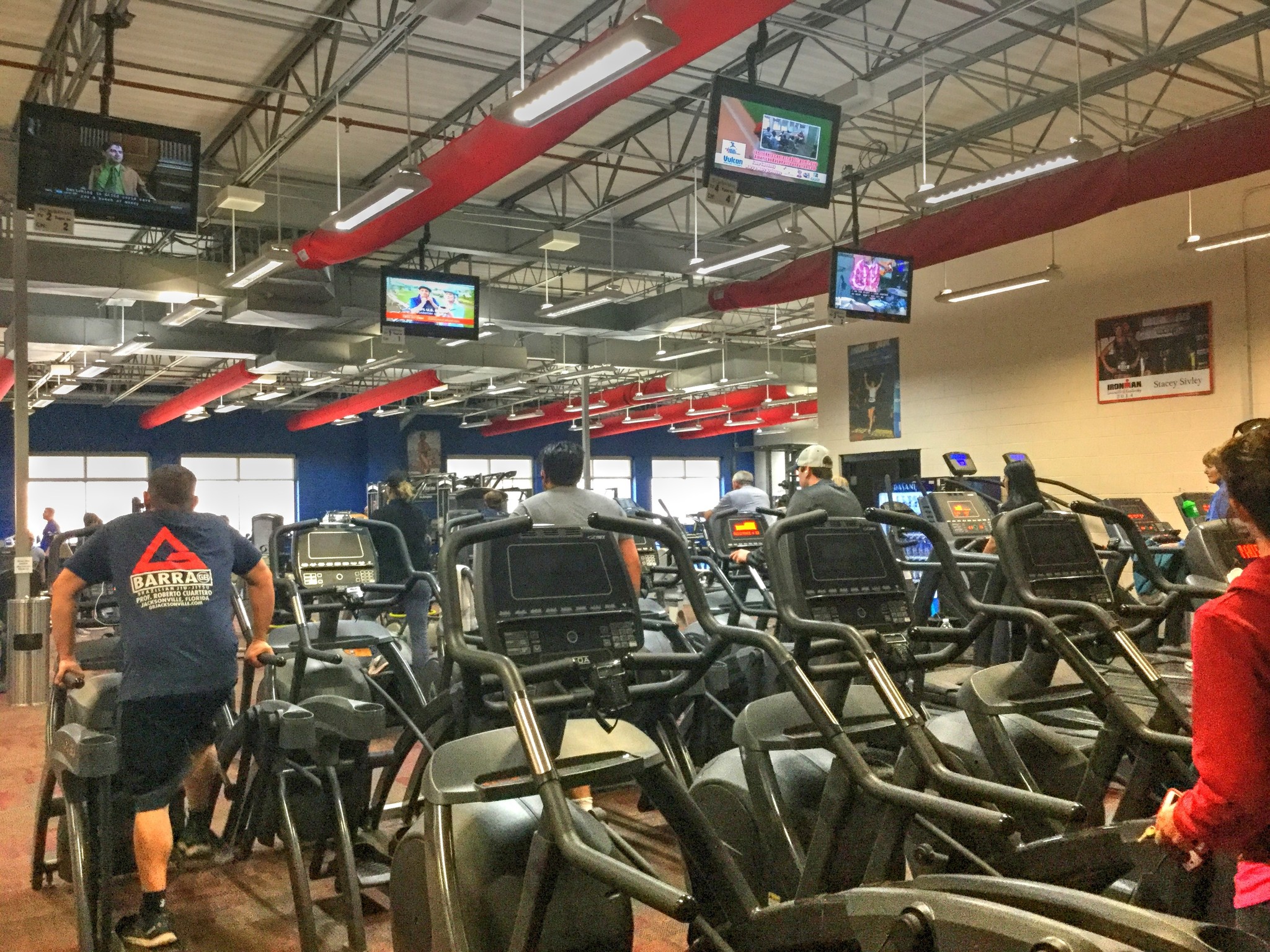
[765,127,804,154]
[29,532,45,586]
[410,283,466,319]
[76,512,104,549]
[864,372,885,434]
[1099,325,1193,378]
[1154,418,1270,943]
[729,444,864,567]
[849,256,905,305]
[510,440,642,818]
[40,508,61,578]
[983,460,1051,554]
[831,476,850,488]
[359,470,436,698]
[1158,446,1230,673]
[86,139,155,200]
[480,490,510,518]
[697,470,772,528]
[50,465,274,948]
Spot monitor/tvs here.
[1208,529,1260,572]
[491,534,631,620]
[938,495,994,536]
[726,517,767,547]
[379,265,481,342]
[1116,503,1155,535]
[20,99,202,232]
[794,524,904,593]
[702,74,842,210]
[1192,498,1213,523]
[827,245,915,325]
[298,527,378,567]
[1018,521,1094,575]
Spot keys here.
[1139,826,1156,842]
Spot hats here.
[384,470,407,488]
[785,445,833,474]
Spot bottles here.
[939,618,954,628]
[1183,500,1199,518]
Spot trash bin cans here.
[6,596,52,707]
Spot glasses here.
[1233,418,1268,438]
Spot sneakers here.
[177,827,212,855]
[114,914,179,947]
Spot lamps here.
[29,0,1270,434]
